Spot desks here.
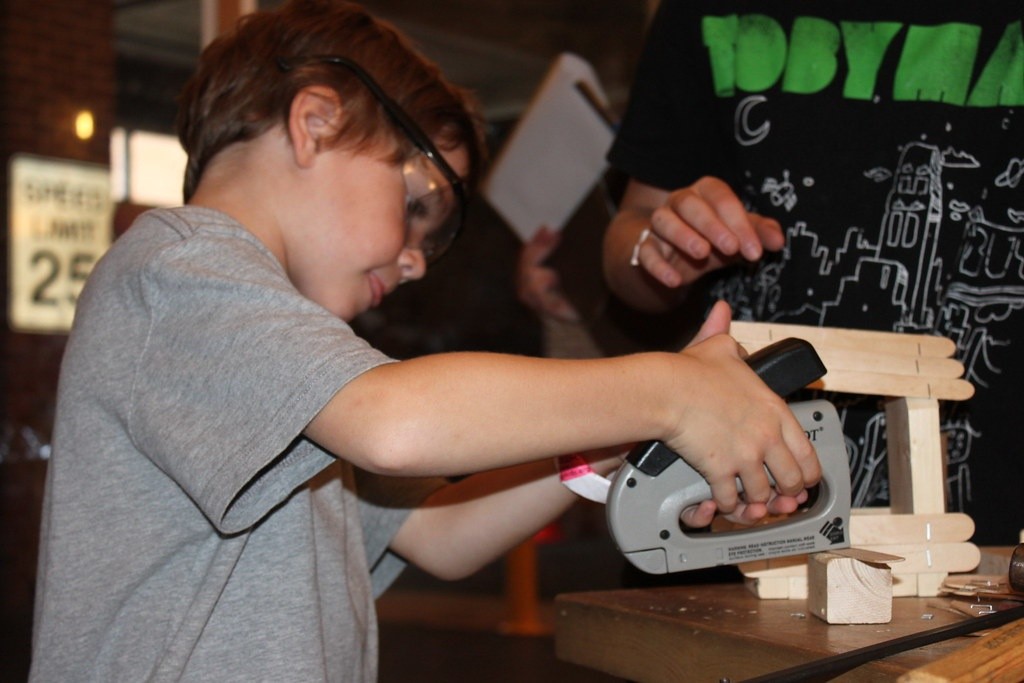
[554,547,1024,683]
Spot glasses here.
[275,54,473,265]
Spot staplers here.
[606,338,852,576]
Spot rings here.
[630,229,650,265]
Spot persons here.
[510,0,1024,556]
[29,0,821,683]
